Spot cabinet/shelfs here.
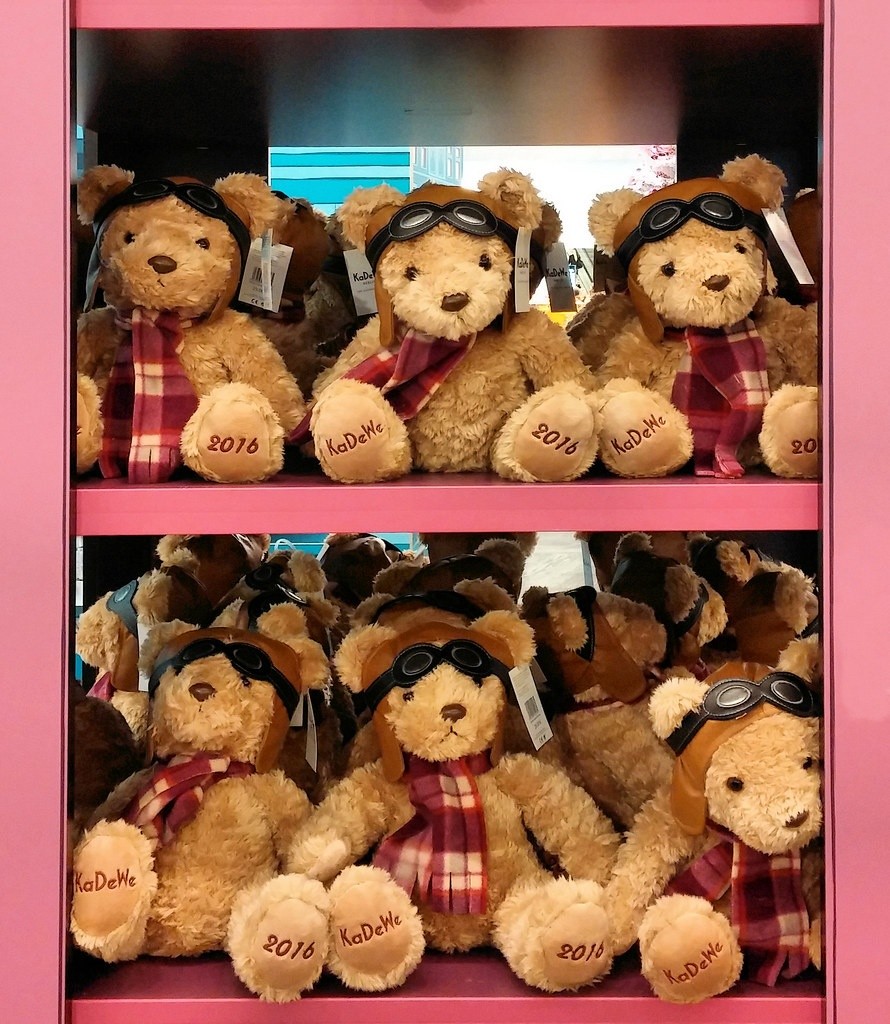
[0,1,890,1024]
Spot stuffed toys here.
[70,530,823,1003]
[76,153,818,484]
